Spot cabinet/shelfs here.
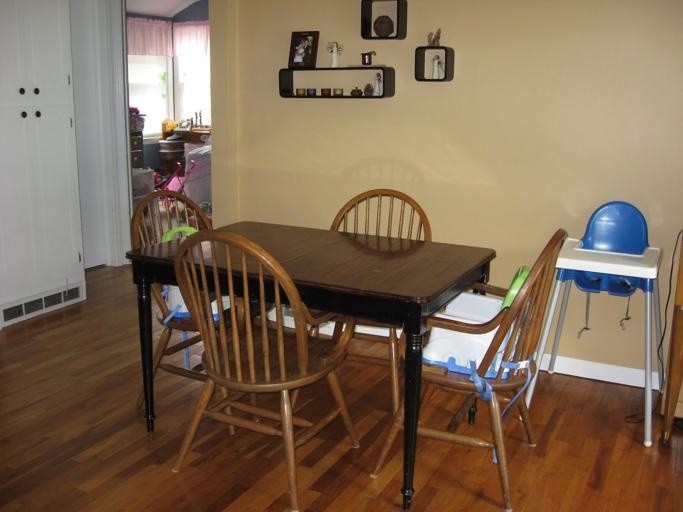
[360,0,408,40]
[0,1,88,328]
[131,132,145,169]
[279,65,395,99]
[414,46,455,81]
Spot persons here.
[433,55,444,79]
[294,38,312,62]
[328,41,342,67]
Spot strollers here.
[154,160,195,213]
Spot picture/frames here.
[288,30,319,69]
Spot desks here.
[125,221,498,512]
